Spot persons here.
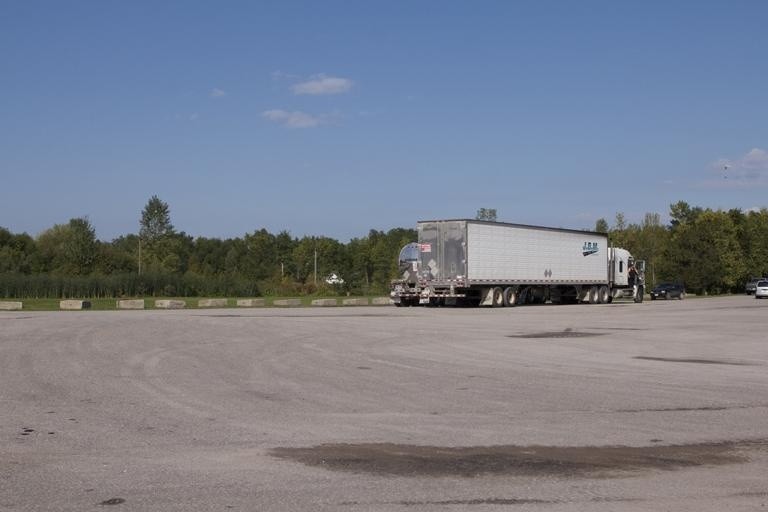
[628,263,639,287]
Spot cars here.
[650,283,685,300]
[745,277,768,295]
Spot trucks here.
[391,241,480,308]
[415,218,647,308]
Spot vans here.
[755,281,768,298]
